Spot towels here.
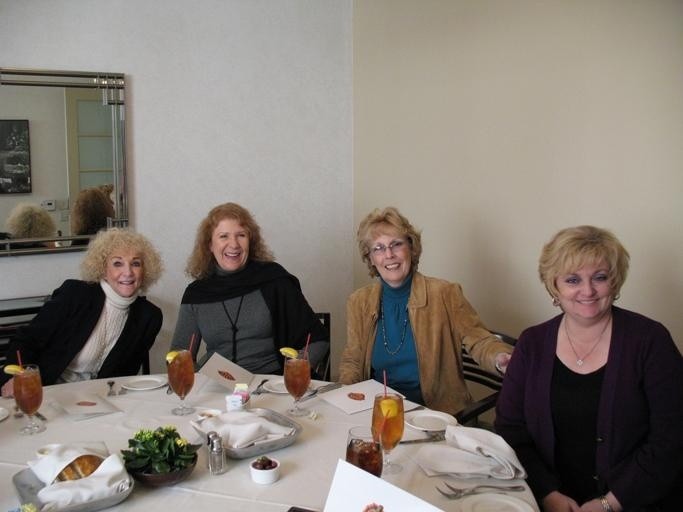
[199,406,294,448]
[421,425,527,484]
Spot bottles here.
[205,432,225,474]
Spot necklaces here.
[380,302,408,355]
[222,295,243,364]
[565,315,612,366]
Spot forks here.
[106,380,118,399]
[432,480,526,500]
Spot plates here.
[264,378,313,394]
[459,492,535,512]
[120,375,168,391]
[0,405,9,422]
[190,407,303,460]
[404,409,456,432]
[12,466,135,512]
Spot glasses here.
[371,242,403,254]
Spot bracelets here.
[496,363,504,374]
[599,495,613,512]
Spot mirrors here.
[1,65,130,259]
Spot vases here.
[129,464,196,488]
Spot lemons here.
[4,364,18,376]
[380,400,397,418]
[280,347,298,359]
[165,352,180,364]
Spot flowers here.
[120,423,202,476]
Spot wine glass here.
[284,350,312,416]
[167,352,199,416]
[371,394,403,475]
[15,364,46,437]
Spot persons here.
[0,226,164,399]
[495,226,683,512]
[69,186,115,246]
[166,202,330,381]
[0,202,57,250]
[338,206,515,428]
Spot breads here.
[56,454,101,482]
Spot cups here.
[249,458,281,484]
[343,426,382,479]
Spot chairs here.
[1,296,153,376]
[309,311,332,383]
[450,327,517,427]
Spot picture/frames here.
[0,119,32,195]
